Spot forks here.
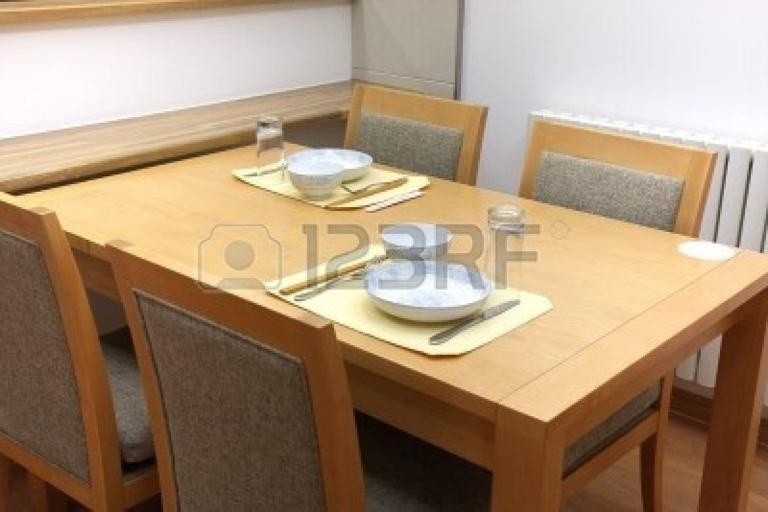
[294,262,380,301]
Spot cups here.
[485,204,527,281]
[256,117,285,185]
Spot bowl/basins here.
[382,223,453,260]
[364,259,490,322]
[286,149,373,181]
[288,158,344,200]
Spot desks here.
[14,140,768,510]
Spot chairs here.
[1,80,721,511]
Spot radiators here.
[527,108,768,415]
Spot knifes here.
[429,298,520,345]
[242,164,286,177]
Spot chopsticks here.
[321,168,407,208]
[279,259,368,296]
